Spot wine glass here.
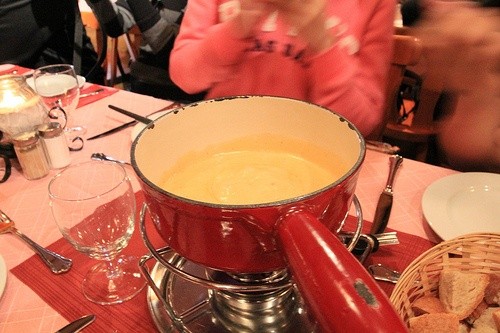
[48,160,150,305]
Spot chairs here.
[386,26,443,162]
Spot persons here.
[407,0,500,171]
[168,0,397,135]
[0,0,79,72]
[83,0,209,105]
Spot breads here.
[408,268,500,333]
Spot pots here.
[130,95,407,333]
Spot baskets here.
[390,232,499,330]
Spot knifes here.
[86,100,182,141]
[56,313,96,333]
[371,156,402,235]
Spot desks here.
[0,63,460,333]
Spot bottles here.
[38,122,72,168]
[13,131,54,180]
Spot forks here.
[0,210,73,275]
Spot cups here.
[0,74,50,137]
[34,64,79,118]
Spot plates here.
[422,172,499,252]
[0,257,8,301]
[27,75,86,96]
